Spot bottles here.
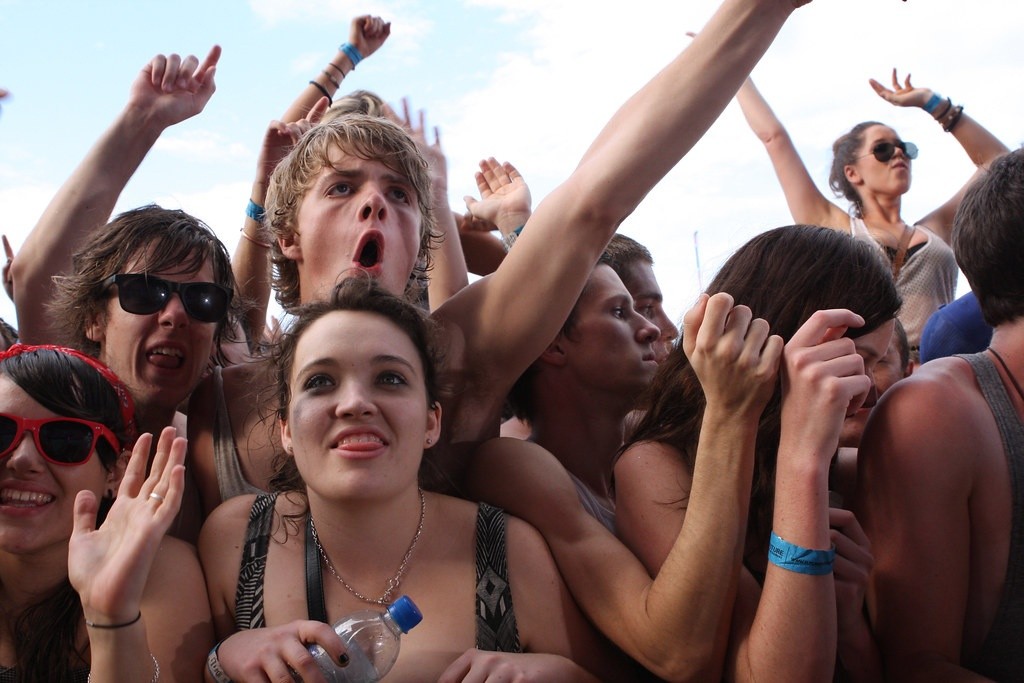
[286,595,423,683]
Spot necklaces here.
[310,486,425,605]
[988,347,1024,401]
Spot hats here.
[919,291,993,364]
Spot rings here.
[149,494,164,501]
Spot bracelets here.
[923,94,941,113]
[330,63,345,78]
[937,98,951,120]
[87,657,160,683]
[241,229,273,248]
[502,226,523,250]
[247,200,265,225]
[945,108,963,132]
[207,641,231,683]
[340,43,362,69]
[321,70,339,90]
[309,81,332,105]
[86,612,140,628]
[768,531,835,575]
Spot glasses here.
[845,142,918,165]
[0,412,120,466]
[98,273,234,322]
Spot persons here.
[205,278,630,683]
[1,0,1024,683]
